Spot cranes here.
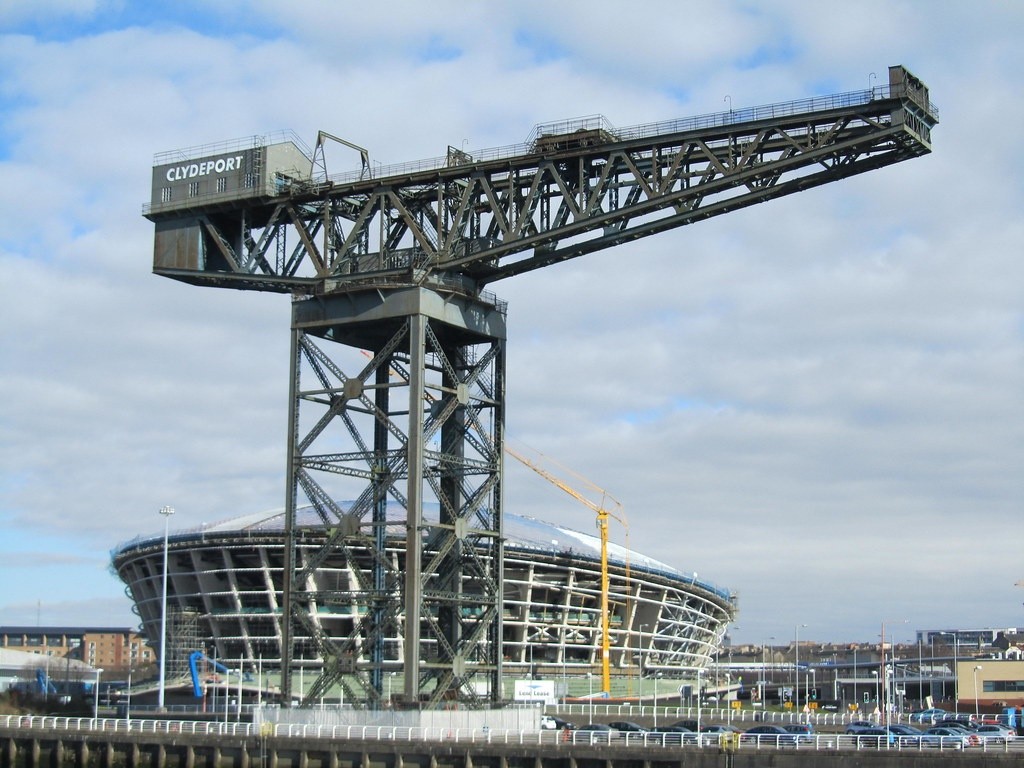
[357,345,633,696]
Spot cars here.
[539,713,814,746]
[845,708,1018,748]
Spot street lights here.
[727,626,742,726]
[91,668,105,729]
[794,624,809,714]
[157,504,176,709]
[638,624,651,712]
[760,635,776,710]
[938,630,957,721]
